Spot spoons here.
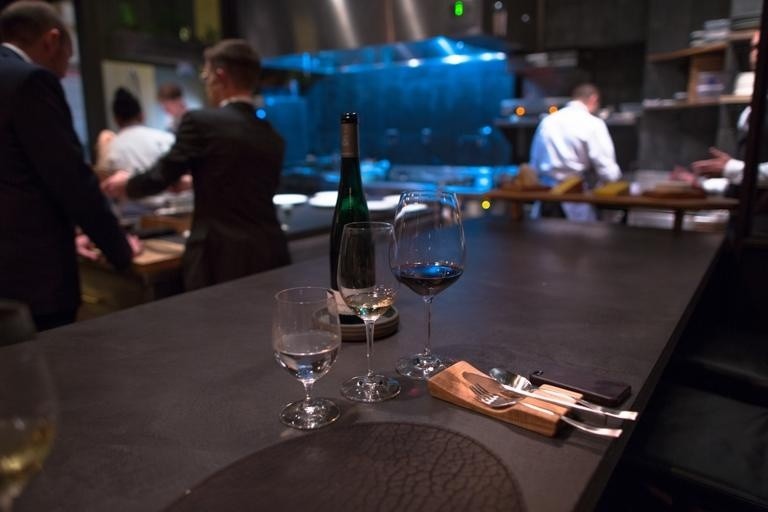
[490,365,602,409]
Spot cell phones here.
[530,363,631,407]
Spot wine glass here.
[337,222,404,403]
[269,283,342,429]
[389,189,468,381]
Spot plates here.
[274,192,401,213]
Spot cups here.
[698,71,724,96]
[1,301,56,509]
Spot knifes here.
[464,370,640,423]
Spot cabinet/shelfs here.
[641,26,756,111]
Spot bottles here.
[331,111,375,322]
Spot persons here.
[158,84,192,193]
[690,31,768,190]
[93,86,176,214]
[1,0,145,330]
[91,126,117,170]
[528,83,624,222]
[98,38,288,302]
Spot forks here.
[468,382,621,442]
